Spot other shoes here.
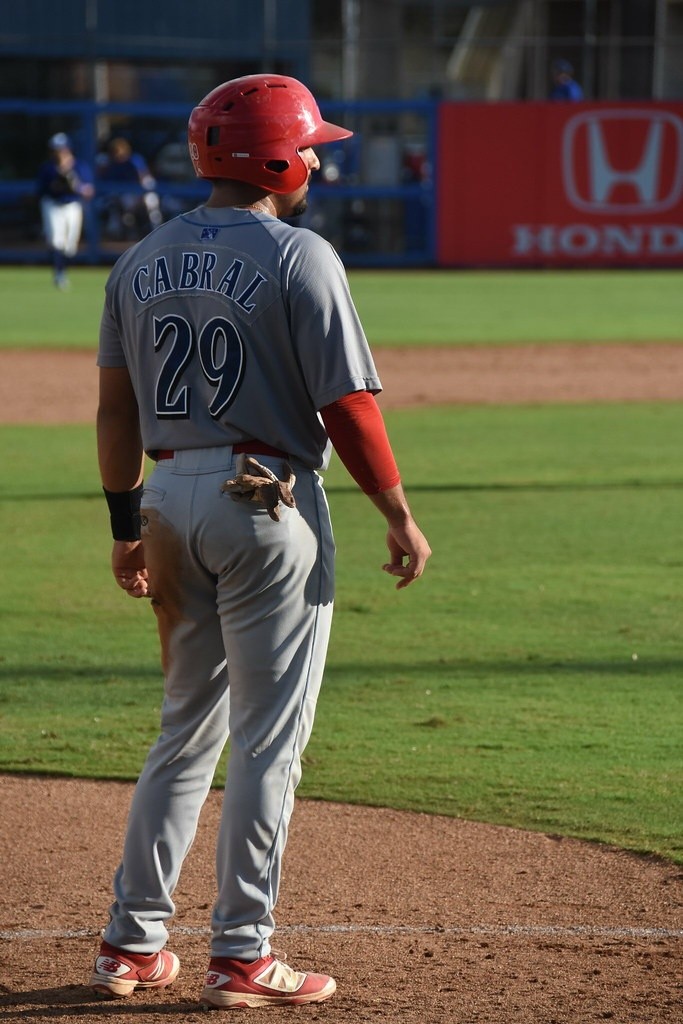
[54,274,67,291]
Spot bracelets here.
[102,480,144,542]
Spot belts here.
[157,441,289,461]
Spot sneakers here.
[198,948,337,1009]
[89,941,180,999]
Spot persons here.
[40,132,163,288]
[91,74,432,1008]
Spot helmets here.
[188,74,354,194]
